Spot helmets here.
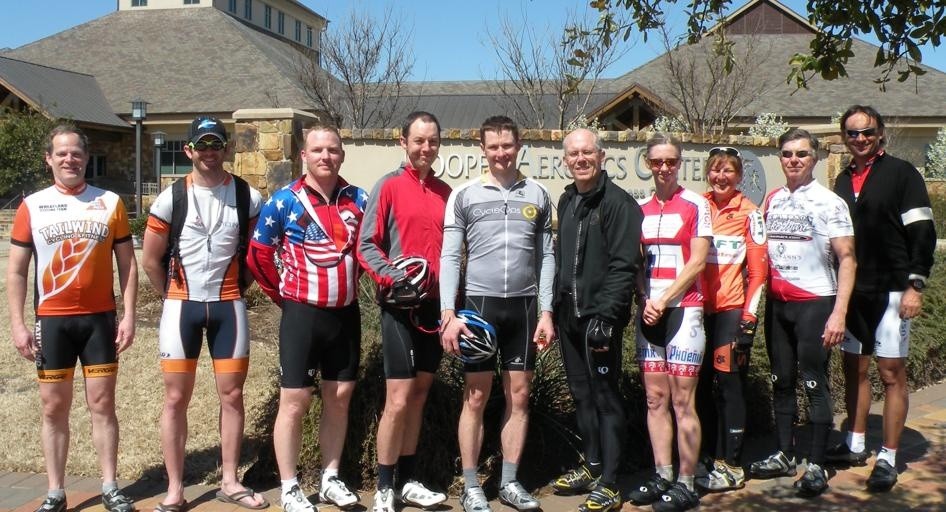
[374,252,439,310]
[435,307,500,366]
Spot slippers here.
[150,492,189,512]
[215,481,271,511]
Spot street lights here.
[151,130,168,197]
[126,96,152,220]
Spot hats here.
[190,113,229,148]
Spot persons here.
[741,128,859,494]
[6,124,138,512]
[245,122,371,511]
[142,117,268,512]
[548,125,644,512]
[691,146,770,491]
[626,132,714,511]
[438,115,557,512]
[823,104,937,492]
[353,111,453,512]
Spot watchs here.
[910,279,926,290]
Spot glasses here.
[780,148,817,160]
[707,146,742,159]
[843,127,881,138]
[645,157,681,168]
[187,137,228,152]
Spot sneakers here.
[371,485,395,512]
[278,480,319,512]
[865,456,901,494]
[548,463,605,495]
[747,449,799,480]
[32,491,68,512]
[99,486,137,512]
[390,475,450,509]
[822,439,870,464]
[457,483,494,512]
[315,471,359,510]
[693,456,747,494]
[626,472,675,506]
[576,482,626,511]
[651,480,701,511]
[790,461,831,496]
[496,478,544,512]
